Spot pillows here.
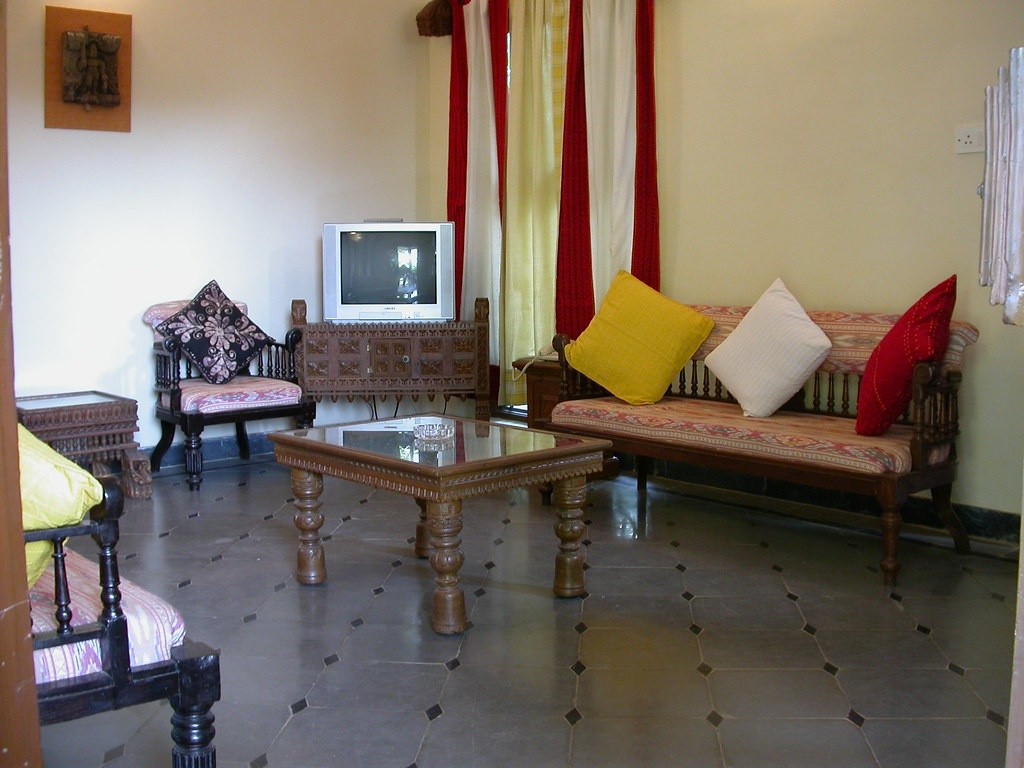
[156,279,277,385]
[703,277,832,417]
[855,272,957,437]
[17,421,104,589]
[565,270,716,407]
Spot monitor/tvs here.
[323,221,456,324]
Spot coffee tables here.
[266,412,612,636]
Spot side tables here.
[513,359,619,482]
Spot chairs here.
[18,478,221,768]
[141,299,316,492]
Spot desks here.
[290,297,489,435]
[15,390,153,500]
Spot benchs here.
[540,304,979,588]
